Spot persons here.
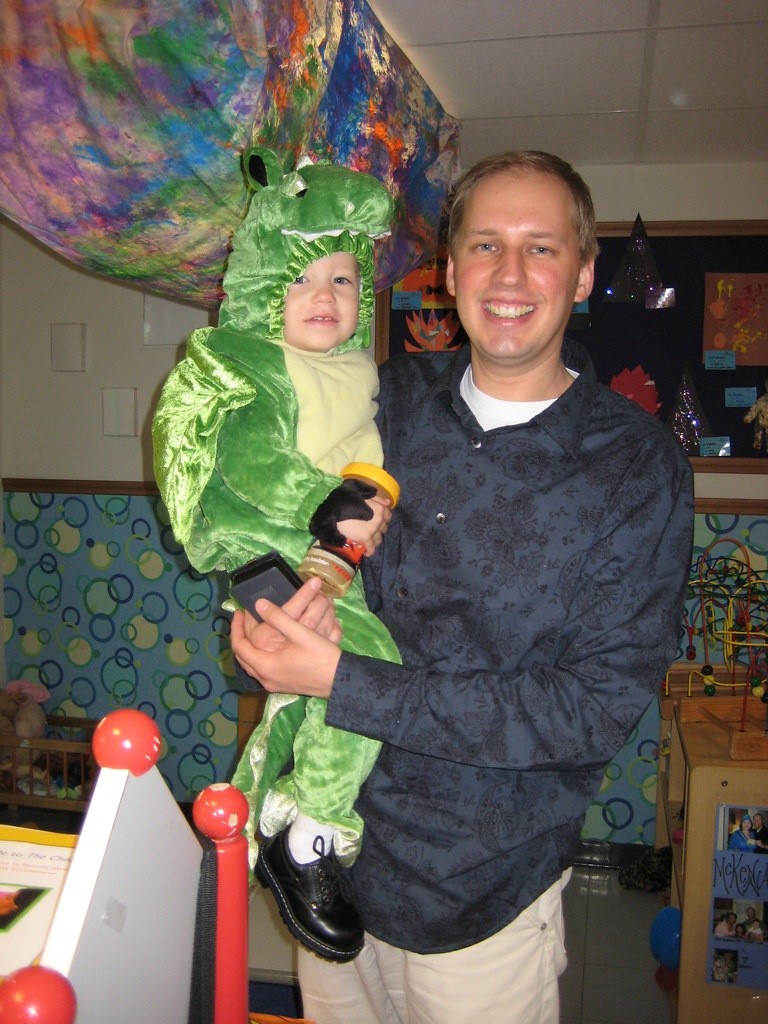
[296,147,691,1024]
[727,813,768,854]
[714,907,768,942]
[152,142,405,966]
[713,951,738,984]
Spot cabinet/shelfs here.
[652,687,768,1024]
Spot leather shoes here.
[255,822,365,965]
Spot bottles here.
[301,464,402,597]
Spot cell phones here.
[231,551,303,591]
